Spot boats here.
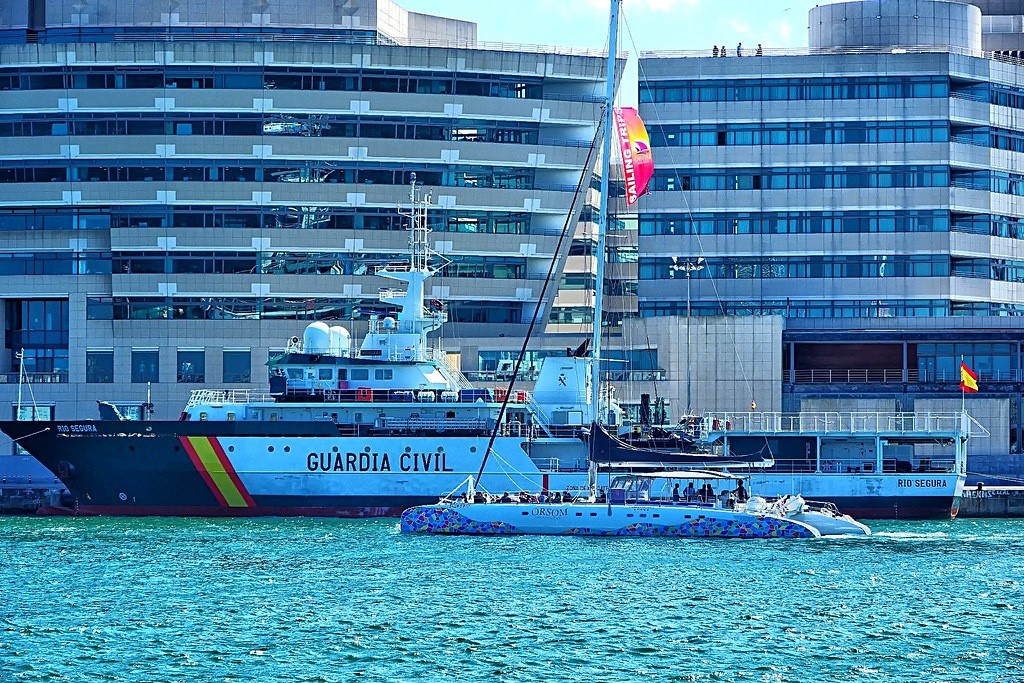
[394,0,869,546]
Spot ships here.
[2,169,992,522]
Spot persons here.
[731,479,750,503]
[720,45,727,57]
[459,490,572,504]
[697,484,710,503]
[755,43,763,57]
[706,483,714,502]
[598,489,606,503]
[712,45,720,58]
[682,481,696,501]
[673,482,681,502]
[736,42,744,57]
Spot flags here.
[613,104,655,206]
[959,360,979,394]
